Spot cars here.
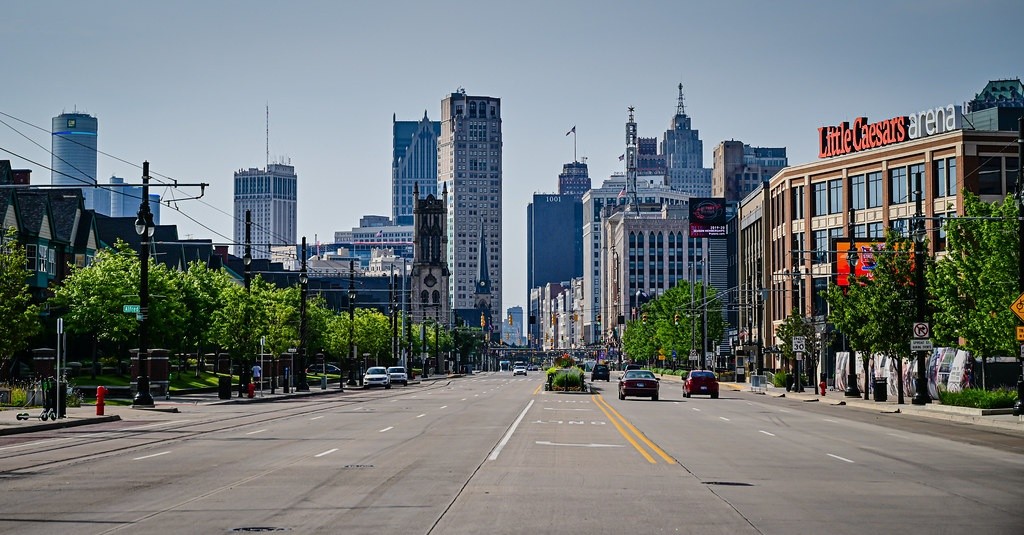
[388,366,408,386]
[363,366,391,390]
[590,364,610,383]
[682,370,719,399]
[618,370,661,401]
[306,363,341,375]
[512,361,543,371]
[624,364,640,373]
[513,365,527,376]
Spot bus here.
[499,360,511,372]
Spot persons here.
[252,363,261,381]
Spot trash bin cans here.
[218,376,232,400]
[872,376,888,402]
[52,383,68,418]
[785,374,793,392]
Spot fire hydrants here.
[819,382,827,396]
[96,386,108,415]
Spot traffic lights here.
[642,313,647,324]
[544,333,549,343]
[675,315,679,326]
[597,315,601,326]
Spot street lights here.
[844,247,861,396]
[389,296,399,367]
[240,246,254,393]
[911,211,932,406]
[406,311,413,380]
[297,273,310,390]
[346,286,357,385]
[133,209,154,405]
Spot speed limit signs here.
[793,336,806,352]
